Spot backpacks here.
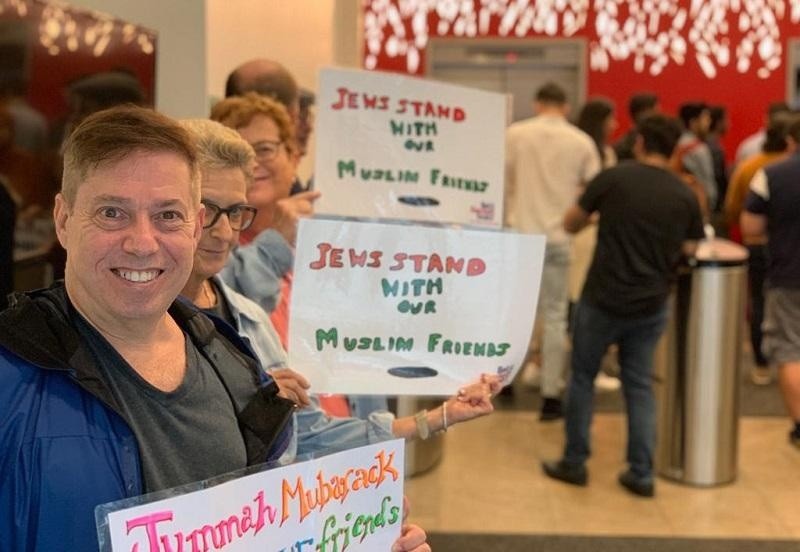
[669,140,700,174]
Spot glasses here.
[251,140,283,163]
[202,203,257,231]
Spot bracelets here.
[443,400,448,430]
[415,409,430,440]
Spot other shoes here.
[752,367,770,385]
[790,426,800,445]
[542,460,588,486]
[540,399,567,420]
[620,470,652,496]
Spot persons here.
[574,94,800,448]
[296,88,314,155]
[0,30,151,303]
[210,93,389,416]
[226,59,300,127]
[540,115,706,502]
[0,103,432,552]
[499,82,602,423]
[173,118,497,459]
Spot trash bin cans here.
[657,238,748,487]
[386,394,444,477]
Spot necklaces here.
[202,284,212,308]
